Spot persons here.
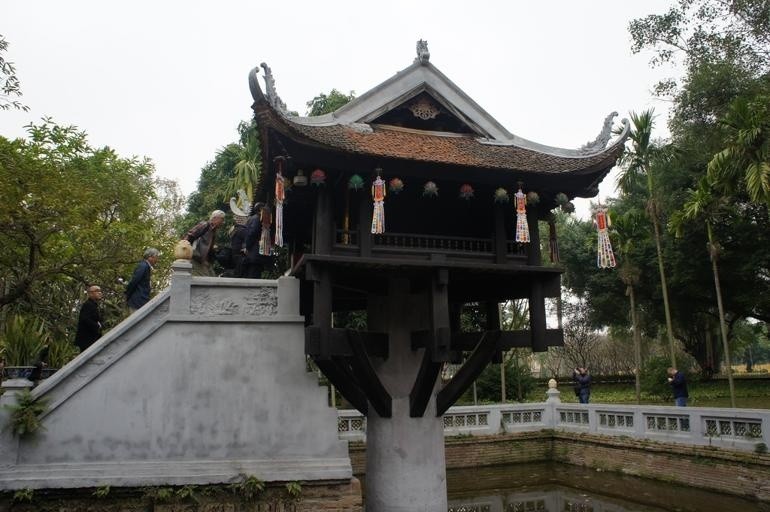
[575,367,591,402]
[124,248,160,315]
[73,286,102,352]
[184,200,270,278]
[667,366,689,429]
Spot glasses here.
[88,289,101,292]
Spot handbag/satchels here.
[187,236,195,245]
